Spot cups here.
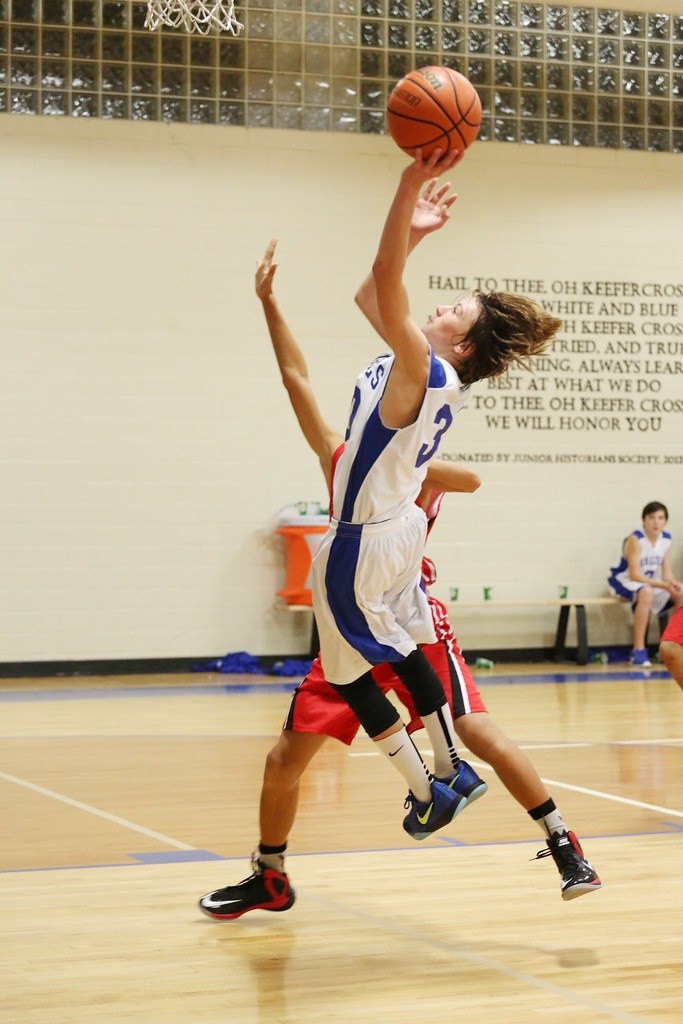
[591,653,607,663]
[450,588,458,600]
[476,658,493,669]
[560,586,567,598]
[484,587,492,600]
[299,502,307,514]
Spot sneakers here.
[653,652,663,662]
[199,857,296,923]
[628,649,652,668]
[401,760,488,841]
[545,830,602,903]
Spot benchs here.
[276,592,675,666]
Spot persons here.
[199,146,600,921]
[606,502,683,689]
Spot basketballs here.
[385,65,483,166]
